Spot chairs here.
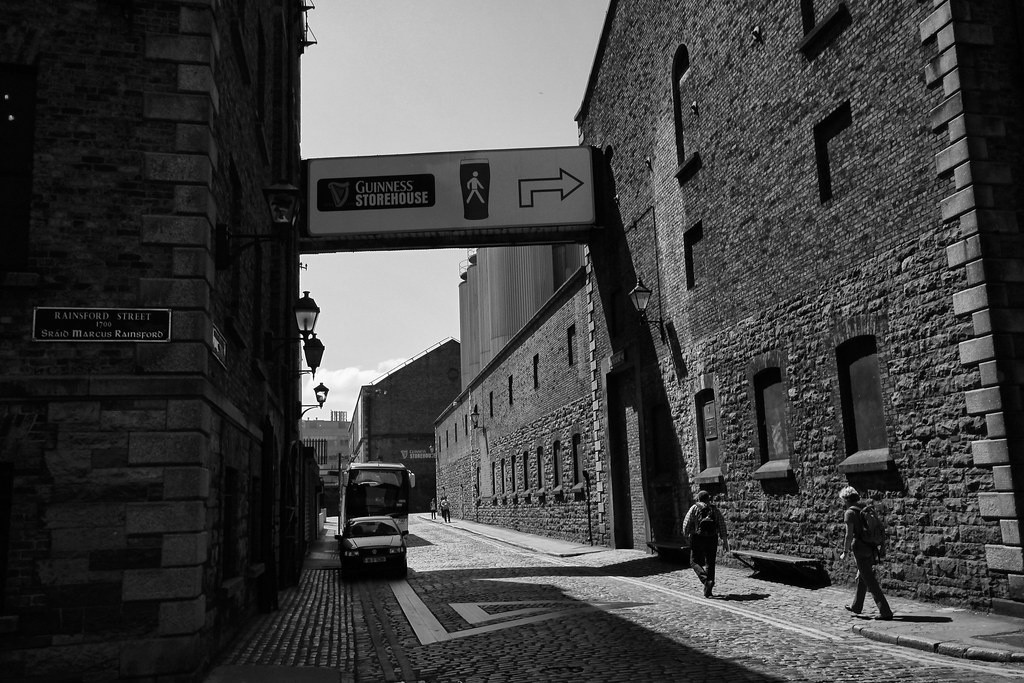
[352,526,363,536]
[376,523,392,534]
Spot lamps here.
[628,276,665,346]
[265,290,320,358]
[469,412,486,433]
[428,445,438,461]
[215,178,302,270]
[297,383,329,420]
[281,334,324,387]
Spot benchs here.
[646,541,693,563]
[730,550,821,585]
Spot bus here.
[340,462,415,542]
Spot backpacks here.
[695,503,717,536]
[850,502,886,549]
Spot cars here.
[335,516,409,579]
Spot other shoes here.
[704,580,714,598]
[875,613,894,620]
[845,604,862,614]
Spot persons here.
[441,496,450,523]
[838,486,893,621]
[430,498,436,519]
[682,491,729,598]
[439,497,445,517]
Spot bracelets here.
[843,551,848,554]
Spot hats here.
[698,491,708,500]
